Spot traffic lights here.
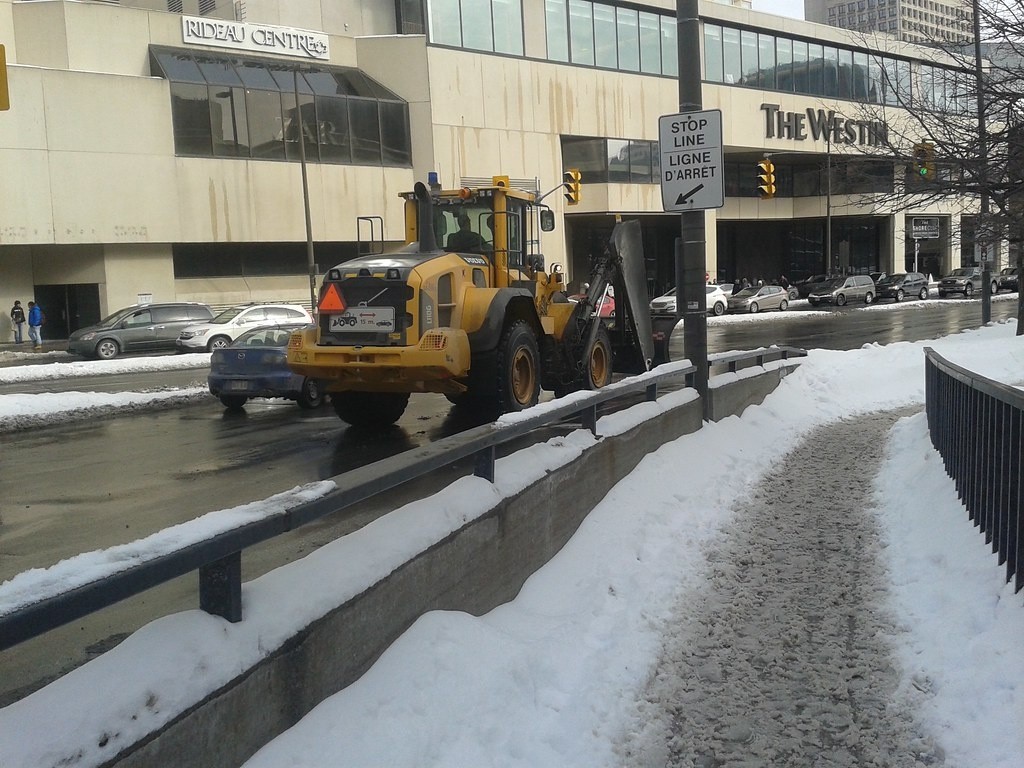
[911,143,936,183]
[757,159,772,199]
[564,168,578,206]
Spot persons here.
[734,277,762,293]
[447,215,493,250]
[11,301,26,344]
[28,302,43,348]
[782,275,789,291]
[708,279,718,285]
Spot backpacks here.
[40,309,48,326]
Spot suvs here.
[807,274,877,306]
[173,301,315,355]
[62,300,218,360]
[875,271,929,301]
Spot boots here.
[32,341,42,349]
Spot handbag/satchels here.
[11,318,18,332]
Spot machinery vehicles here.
[286,175,682,427]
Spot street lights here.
[214,86,242,158]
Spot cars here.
[937,266,1000,296]
[726,286,790,314]
[784,284,799,301]
[868,270,888,285]
[649,284,728,317]
[206,322,334,411]
[564,294,616,316]
[999,266,1022,291]
[796,274,829,297]
[716,283,735,299]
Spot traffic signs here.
[658,107,726,213]
